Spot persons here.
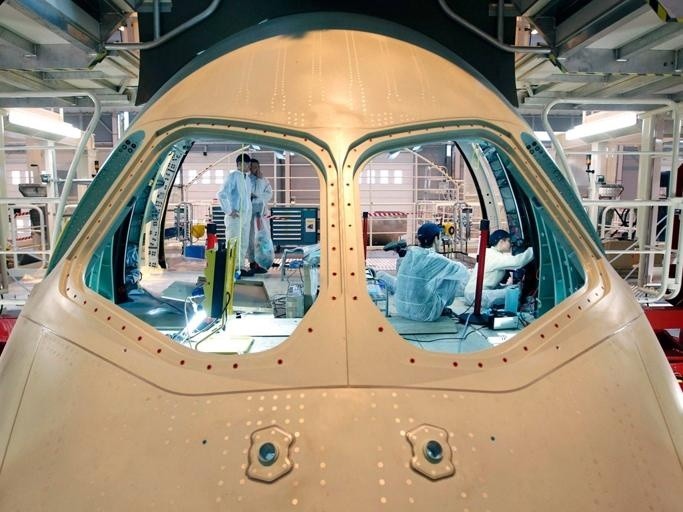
[463,229,535,308]
[216,153,254,277]
[393,223,467,321]
[249,159,275,272]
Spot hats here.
[490,230,516,246]
[417,223,443,242]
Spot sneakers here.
[382,239,407,257]
[440,307,453,316]
[237,261,267,277]
